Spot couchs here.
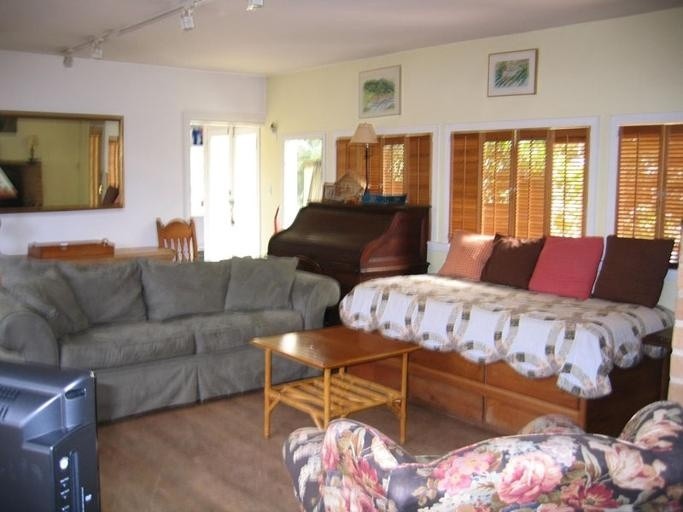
[0,256,340,424]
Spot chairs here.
[156,218,199,262]
[282,400,683,512]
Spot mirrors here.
[0,110,125,213]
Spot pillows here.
[590,236,675,308]
[439,231,493,281]
[138,258,234,324]
[224,257,301,312]
[479,232,546,290]
[56,261,147,325]
[1,265,91,332]
[528,235,605,299]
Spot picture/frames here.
[486,48,539,98]
[358,64,402,119]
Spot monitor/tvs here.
[1,358,101,512]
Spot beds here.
[331,273,679,436]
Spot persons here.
[0,168,18,202]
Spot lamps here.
[61,0,265,68]
[350,123,380,193]
[24,134,40,164]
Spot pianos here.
[268,202,429,328]
